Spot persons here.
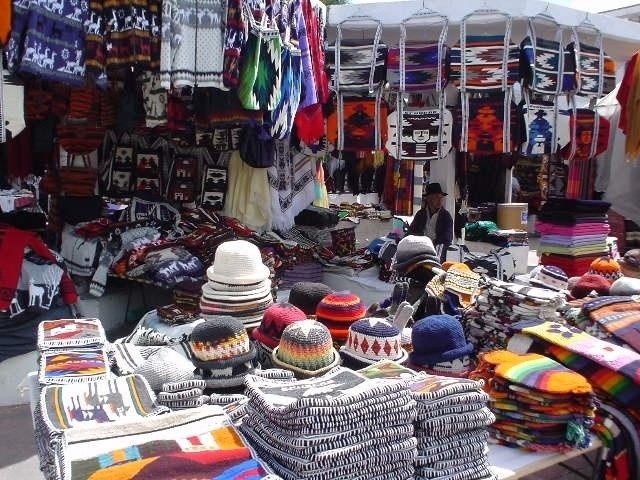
[404,182,455,266]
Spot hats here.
[422,182,448,197]
[191,317,258,368]
[529,248,640,299]
[316,291,366,341]
[391,235,443,275]
[288,282,333,322]
[252,302,309,348]
[198,241,273,330]
[366,235,473,321]
[272,319,339,379]
[191,359,262,388]
[339,317,408,366]
[409,314,472,378]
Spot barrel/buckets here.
[495,202,529,232]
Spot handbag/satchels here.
[239,119,277,168]
[30,111,242,226]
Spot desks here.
[485,430,604,480]
[109,273,169,325]
